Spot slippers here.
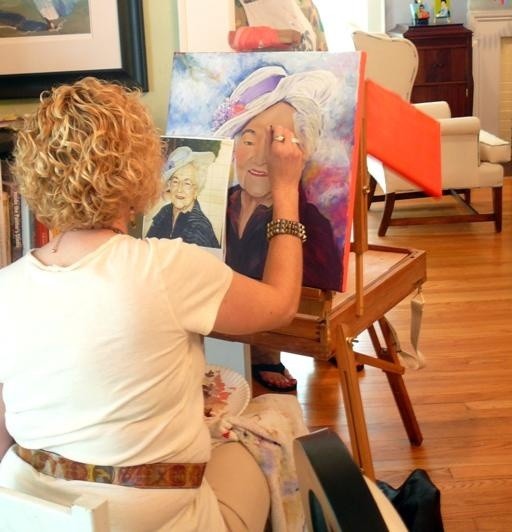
[251,362,297,391]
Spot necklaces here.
[48,226,124,234]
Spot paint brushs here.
[274,137,301,143]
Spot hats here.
[158,145,216,202]
[210,65,345,140]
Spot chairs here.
[353,30,512,237]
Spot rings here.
[292,138,301,144]
[273,136,284,141]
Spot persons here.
[0,75,311,530]
[145,145,220,247]
[417,4,430,19]
[436,0,450,19]
[227,0,364,392]
[206,62,342,292]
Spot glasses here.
[169,177,198,190]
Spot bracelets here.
[265,219,308,244]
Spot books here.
[0,183,48,265]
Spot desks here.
[388,24,473,119]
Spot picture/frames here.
[0,1,148,100]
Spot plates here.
[204,363,251,419]
